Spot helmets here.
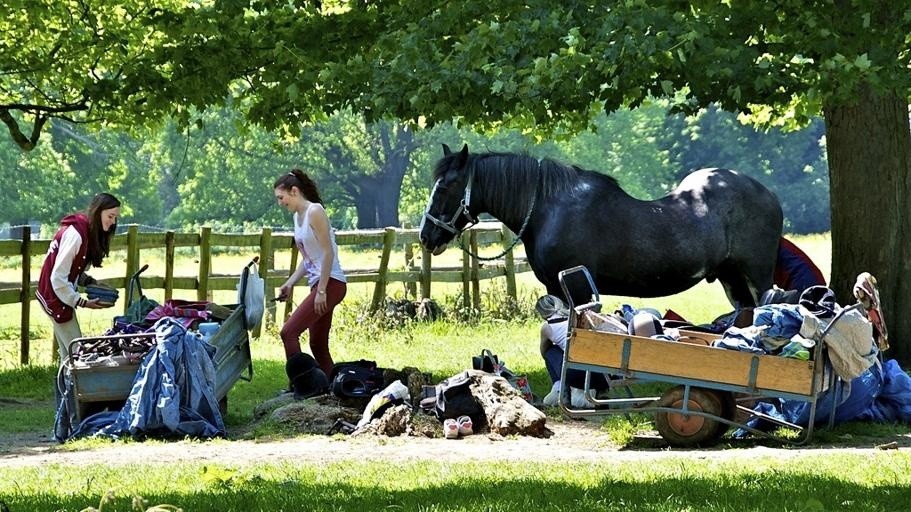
[286,352,329,399]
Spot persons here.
[771,237,827,294]
[35,193,120,365]
[274,169,347,375]
[536,295,610,390]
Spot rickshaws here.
[547,266,863,449]
[69,256,257,424]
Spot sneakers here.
[543,382,570,406]
[443,419,459,438]
[457,416,473,436]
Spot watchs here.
[317,290,327,294]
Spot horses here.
[416,141,784,309]
